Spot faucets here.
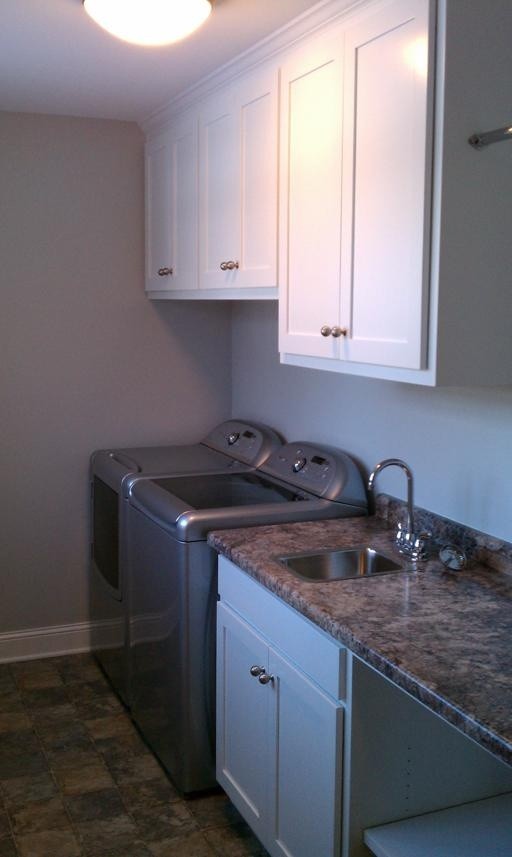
[365,457,416,554]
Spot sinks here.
[272,544,406,583]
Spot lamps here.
[78,0,213,44]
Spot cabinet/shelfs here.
[195,53,282,301]
[211,555,509,857]
[139,105,199,302]
[273,0,512,391]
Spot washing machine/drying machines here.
[124,416,371,799]
[87,415,287,800]
